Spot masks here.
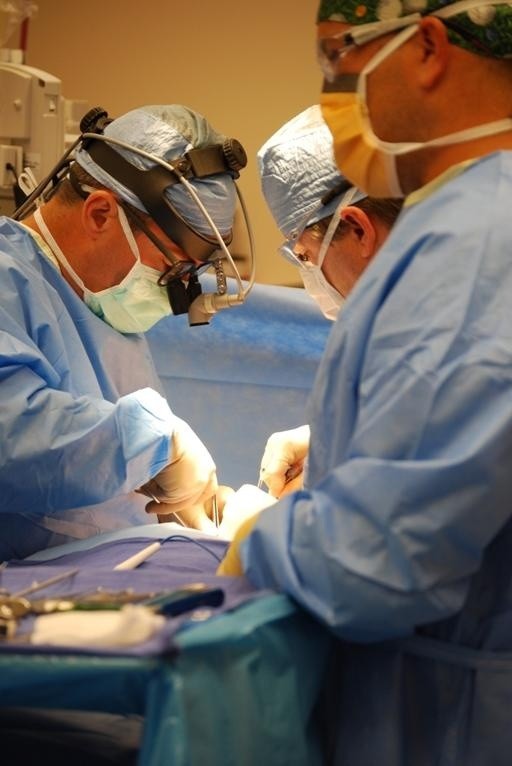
[296,207,346,322]
[319,16,422,201]
[82,202,188,337]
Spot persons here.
[1,100,249,560]
[215,1,510,764]
[257,101,401,502]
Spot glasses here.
[314,11,475,86]
[76,180,215,286]
[277,179,353,273]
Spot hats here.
[70,103,237,237]
[254,104,370,237]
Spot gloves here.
[135,415,235,533]
[220,425,310,543]
